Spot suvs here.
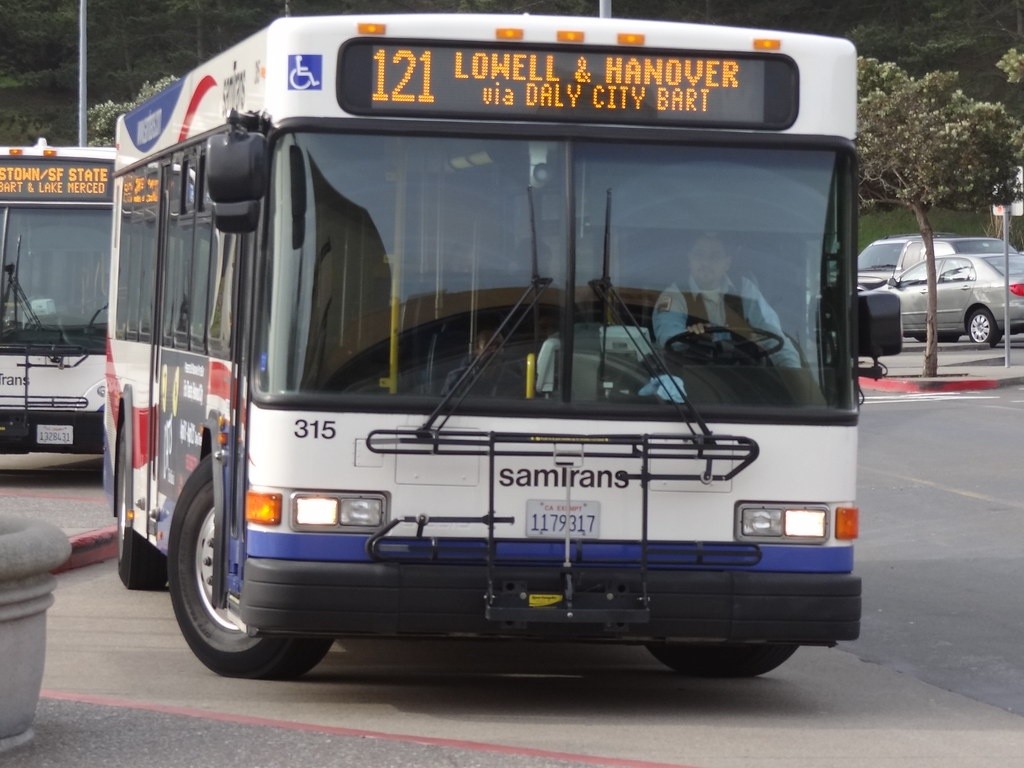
[847,230,1018,295]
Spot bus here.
[1,136,197,457]
[102,11,904,682]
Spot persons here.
[652,231,828,408]
[440,328,531,397]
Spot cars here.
[853,250,1024,348]
